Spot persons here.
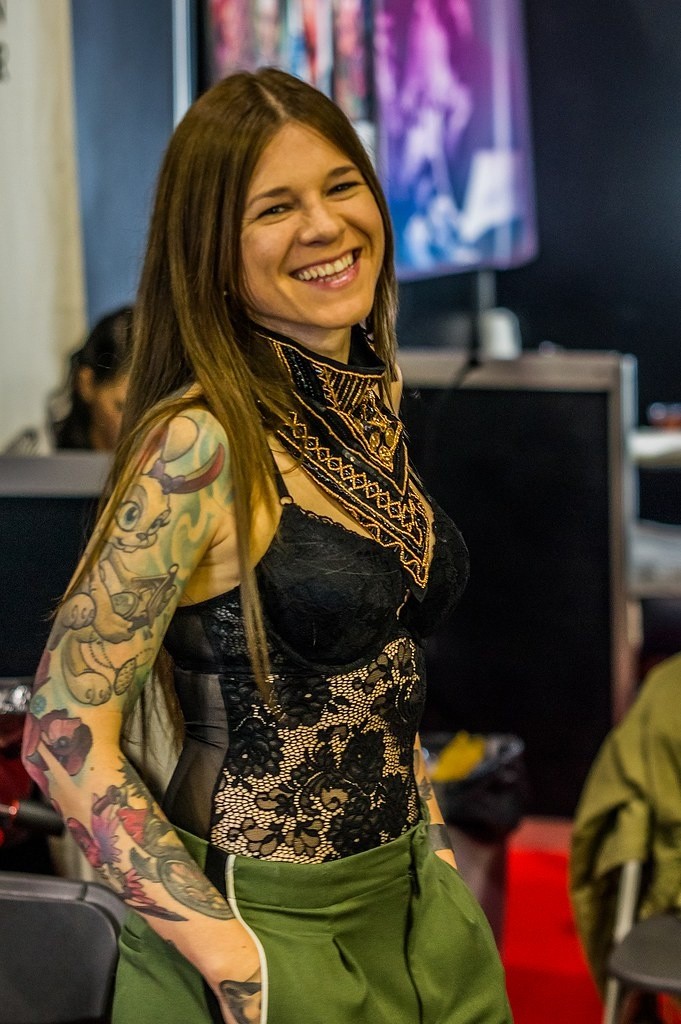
[248,0,282,66]
[283,0,332,95]
[4,308,135,462]
[330,0,371,120]
[19,64,515,1024]
[208,0,253,88]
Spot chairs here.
[0,869,124,1024]
[568,653,681,1024]
